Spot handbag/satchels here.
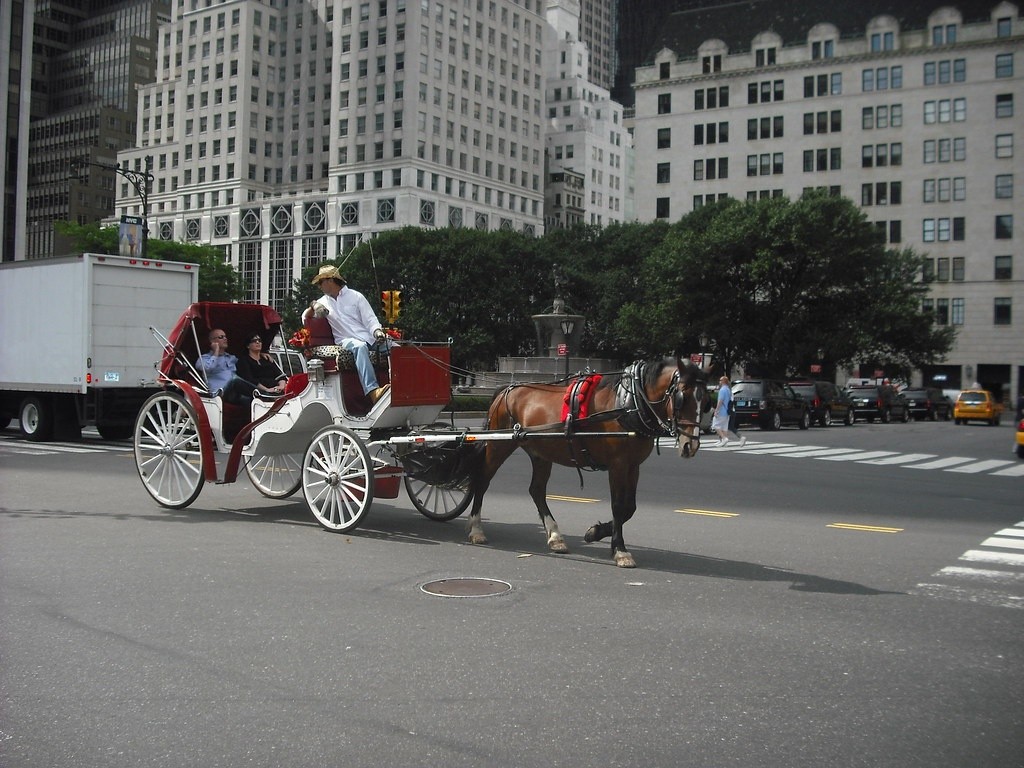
[727,400,734,415]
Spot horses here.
[465,358,717,569]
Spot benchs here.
[303,308,402,369]
[189,375,252,440]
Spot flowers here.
[288,329,312,359]
[388,328,400,339]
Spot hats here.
[311,264,347,285]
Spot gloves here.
[374,329,388,345]
[310,300,329,319]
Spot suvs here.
[898,387,954,421]
[787,380,858,427]
[847,385,910,423]
[953,390,1003,426]
[730,377,812,431]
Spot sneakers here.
[370,384,390,405]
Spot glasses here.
[318,278,328,285]
[250,338,263,342]
[210,335,228,340]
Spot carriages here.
[132,299,716,568]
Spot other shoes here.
[720,437,729,447]
[739,437,746,447]
[715,441,721,445]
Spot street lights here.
[559,313,575,387]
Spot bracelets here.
[273,387,278,392]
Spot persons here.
[711,376,746,448]
[302,265,400,404]
[196,329,273,405]
[552,264,563,297]
[235,332,288,400]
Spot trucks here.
[0,250,201,442]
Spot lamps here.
[965,365,973,379]
